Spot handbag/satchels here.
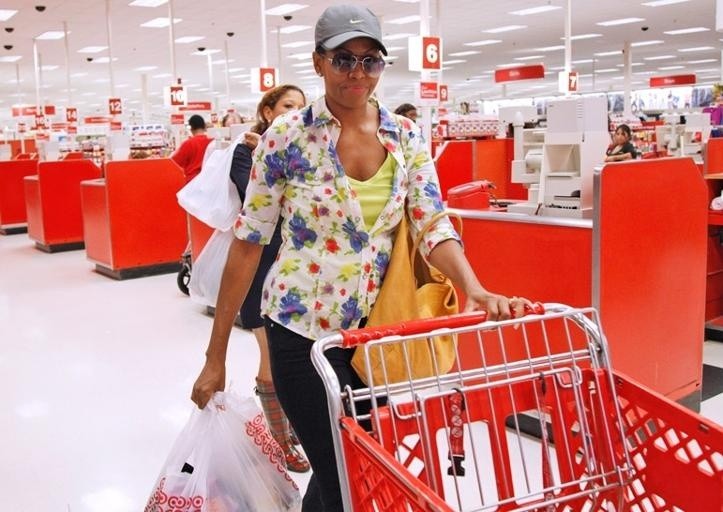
[350,207,464,397]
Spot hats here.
[314,4,389,57]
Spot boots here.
[254,377,311,473]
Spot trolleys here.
[178,243,192,298]
[309,302,722,512]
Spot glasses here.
[321,53,386,78]
